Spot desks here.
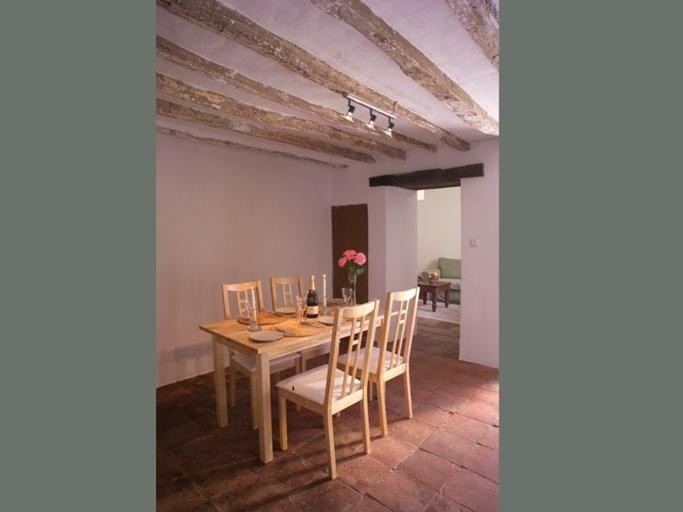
[418,281,452,312]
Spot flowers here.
[335,245,367,288]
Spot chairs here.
[274,298,382,481]
[333,285,421,439]
[218,277,304,430]
[268,270,333,372]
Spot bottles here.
[307,275,318,319]
[242,286,257,329]
[417,271,438,283]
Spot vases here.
[349,281,356,306]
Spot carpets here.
[416,296,460,325]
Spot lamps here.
[338,92,398,141]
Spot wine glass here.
[342,287,352,305]
[296,295,307,322]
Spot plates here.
[327,299,344,304]
[318,316,346,324]
[275,306,296,315]
[248,330,282,341]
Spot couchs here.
[421,256,461,306]
